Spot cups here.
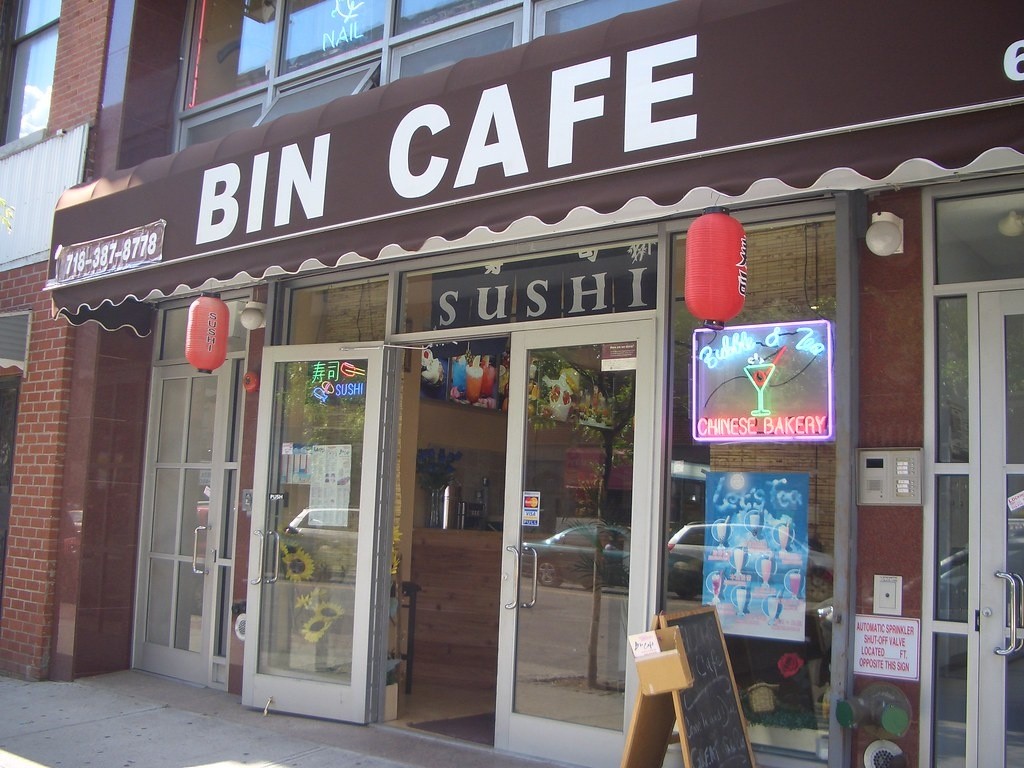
[480,366,495,398]
[452,361,469,400]
[466,367,483,404]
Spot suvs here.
[671,519,834,602]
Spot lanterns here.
[185,291,230,374]
[684,206,748,330]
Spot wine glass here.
[711,576,721,602]
[789,573,801,600]
[761,560,771,586]
[779,527,789,551]
[750,515,760,537]
[717,523,726,549]
[733,550,744,575]
[736,589,747,617]
[768,598,778,625]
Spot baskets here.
[749,680,774,713]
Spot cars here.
[518,525,632,591]
[282,507,359,581]
[809,536,1023,742]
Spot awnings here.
[42,0,1024,337]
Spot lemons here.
[472,355,481,368]
[483,355,489,366]
[459,355,466,364]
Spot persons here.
[604,537,618,551]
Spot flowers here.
[416,445,464,492]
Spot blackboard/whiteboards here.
[619,604,757,768]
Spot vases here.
[425,489,444,528]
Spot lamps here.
[239,301,268,330]
[865,210,905,258]
